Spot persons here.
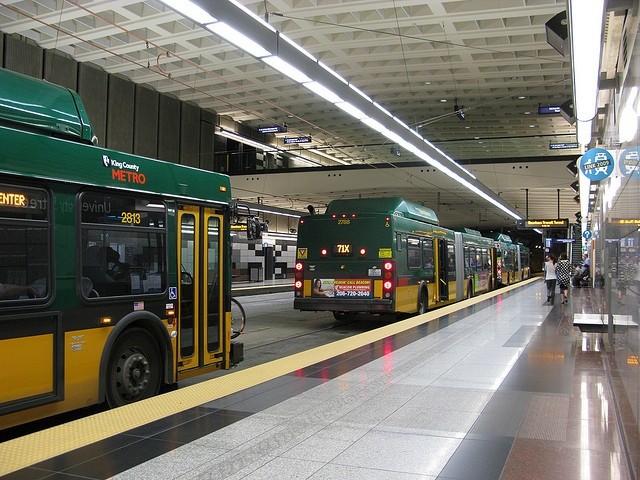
[544,252,558,305]
[542,254,549,284]
[428,259,434,268]
[556,252,571,304]
[424,261,429,268]
[615,253,640,306]
[571,254,591,288]
[313,279,329,297]
[448,258,454,267]
[595,260,605,289]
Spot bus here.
[488,233,531,286]
[1,65,262,431]
[294,195,501,320]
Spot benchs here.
[573,312,602,334]
[604,314,638,333]
